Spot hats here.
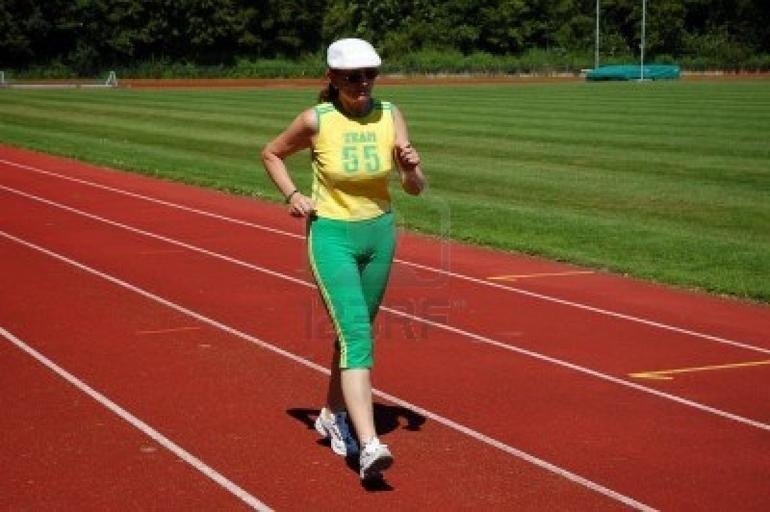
[326,37,381,70]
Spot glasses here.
[335,68,379,83]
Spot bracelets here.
[286,190,298,203]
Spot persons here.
[262,36,426,485]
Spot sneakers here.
[313,405,358,456]
[360,435,394,481]
